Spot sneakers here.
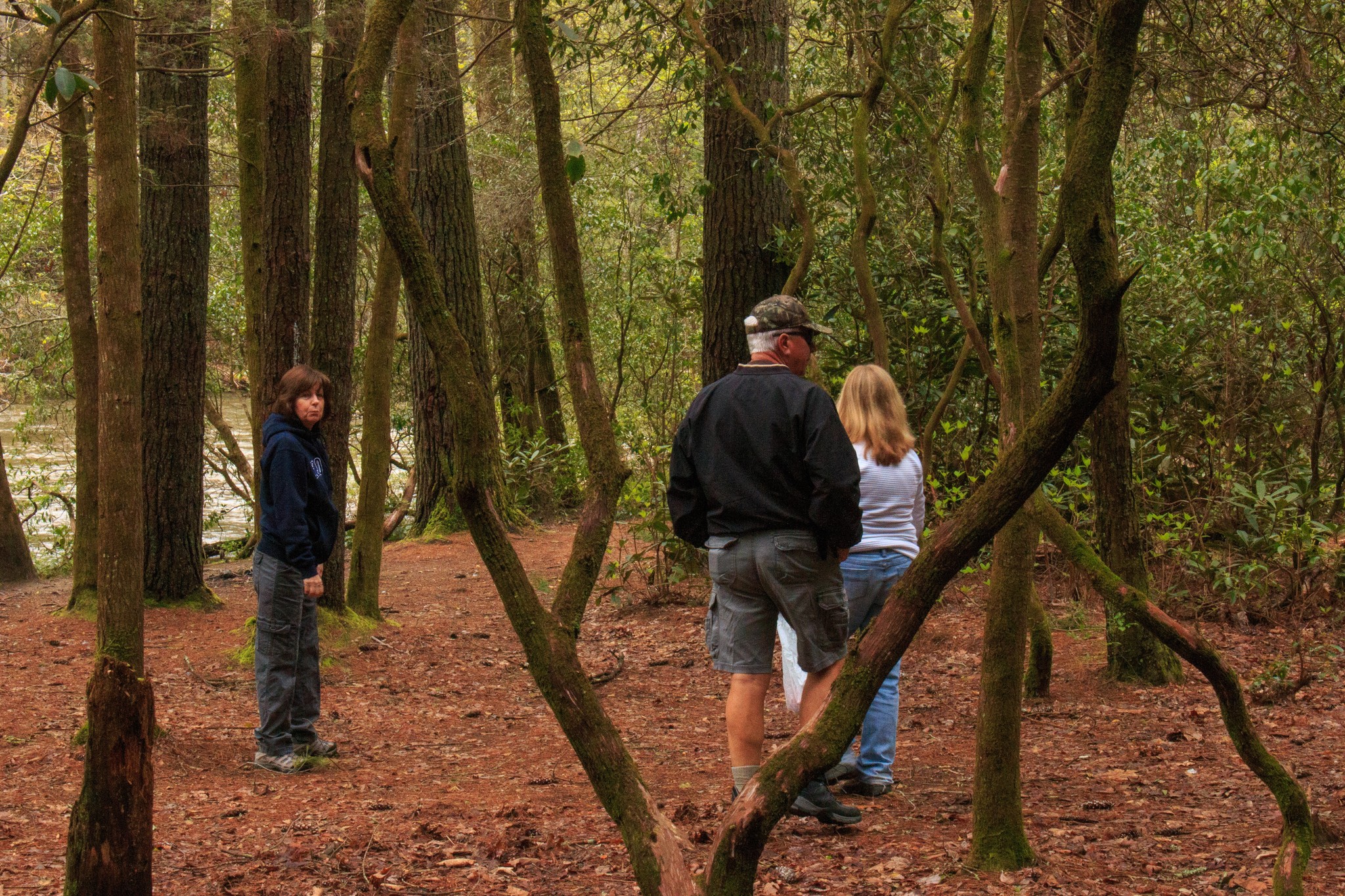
[731,785,741,803]
[254,750,314,774]
[820,764,857,795]
[790,772,863,824]
[838,778,893,796]
[294,736,338,758]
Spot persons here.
[667,295,861,824]
[254,366,341,774]
[829,363,926,793]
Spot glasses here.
[772,331,814,347]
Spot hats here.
[744,294,833,337]
